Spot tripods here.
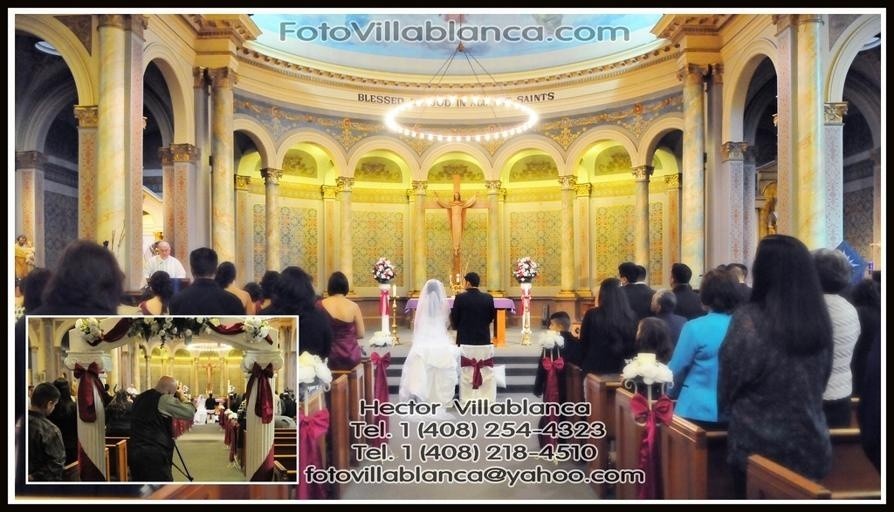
[169,437,196,482]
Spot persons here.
[531,232,880,501]
[396,280,457,422]
[14,235,365,499]
[448,271,495,346]
[435,188,478,256]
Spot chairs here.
[207,410,215,423]
[457,342,497,404]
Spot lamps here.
[382,14,541,143]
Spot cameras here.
[174,388,182,401]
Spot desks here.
[404,298,517,346]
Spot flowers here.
[371,256,397,284]
[224,408,233,415]
[369,330,395,348]
[623,352,674,385]
[511,256,538,283]
[297,352,334,384]
[228,413,238,419]
[218,405,225,409]
[75,317,270,345]
[538,330,565,348]
